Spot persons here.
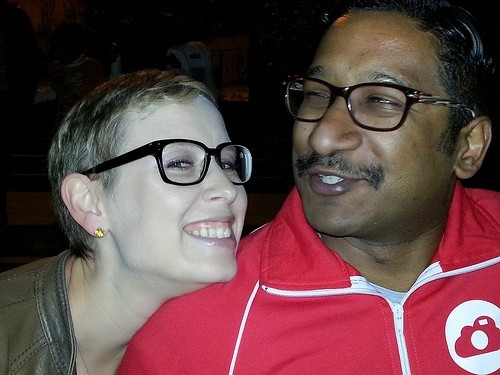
[112,0,499,375]
[0,68,253,375]
[0,0,188,191]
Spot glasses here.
[281,74,476,133]
[80,138,254,186]
